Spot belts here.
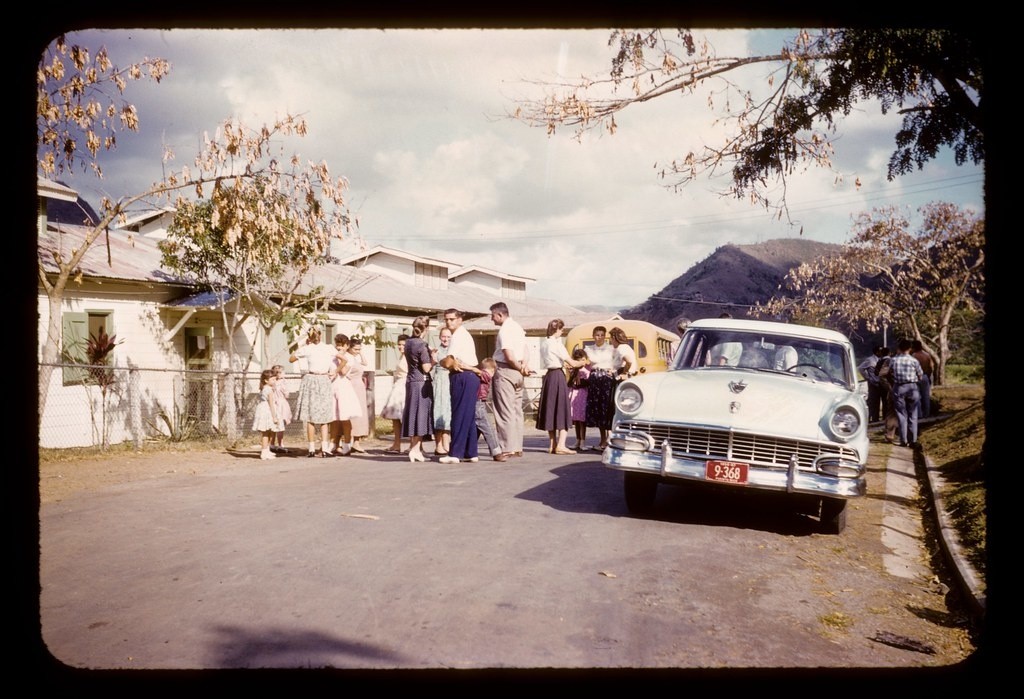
[308,372,327,375]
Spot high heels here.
[409,449,432,463]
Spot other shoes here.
[549,447,577,455]
[260,450,276,460]
[909,441,918,449]
[434,448,450,455]
[568,443,580,449]
[502,450,523,458]
[599,442,607,449]
[439,455,460,464]
[270,445,288,453]
[340,446,352,456]
[899,443,907,447]
[579,445,589,451]
[352,447,365,453]
[493,452,507,462]
[461,456,479,462]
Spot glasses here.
[351,347,361,350]
[444,318,459,321]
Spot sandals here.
[404,448,411,453]
[383,447,400,453]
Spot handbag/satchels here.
[566,366,587,390]
[878,360,891,376]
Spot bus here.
[562,316,681,395]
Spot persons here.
[403,316,438,462]
[433,327,452,455]
[859,340,932,448]
[380,334,411,454]
[711,313,742,367]
[667,317,711,368]
[535,318,590,454]
[460,358,507,462]
[774,344,798,373]
[289,326,369,458]
[489,302,531,458]
[439,309,480,464]
[252,365,292,460]
[568,326,636,450]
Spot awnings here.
[159,286,279,341]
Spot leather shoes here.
[323,451,335,457]
[308,451,315,457]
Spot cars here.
[598,316,872,536]
